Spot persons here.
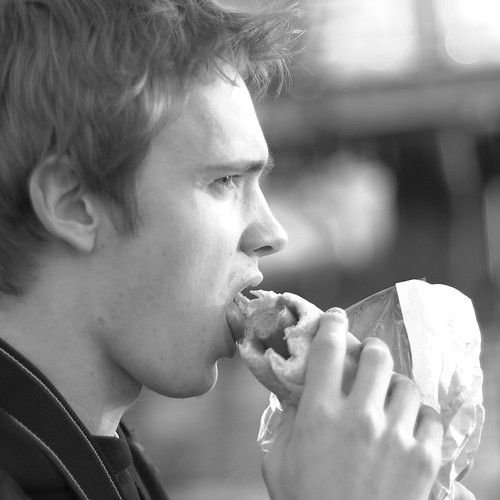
[0,1,444,496]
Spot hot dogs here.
[229,289,474,499]
